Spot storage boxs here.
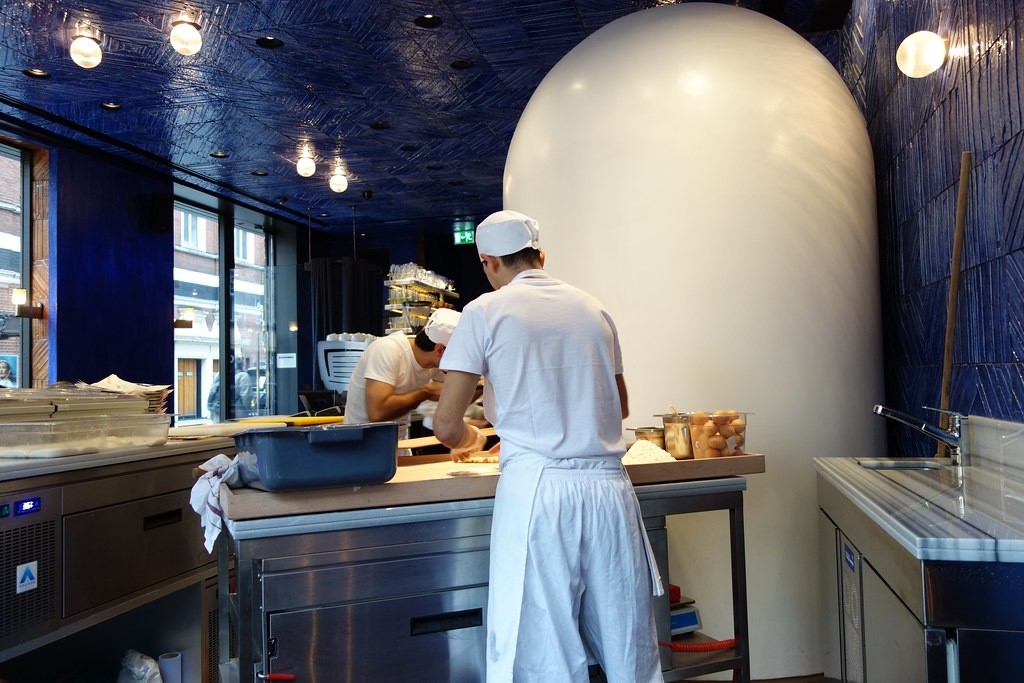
[229,418,409,491]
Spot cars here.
[246,365,267,417]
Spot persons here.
[0,360,16,389]
[433,211,667,683]
[344,308,461,457]
[210,356,253,423]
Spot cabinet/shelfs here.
[383,279,459,336]
[817,473,1024,683]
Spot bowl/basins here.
[326,332,375,344]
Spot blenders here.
[404,300,432,337]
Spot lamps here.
[170,21,202,56]
[11,288,42,320]
[329,174,348,193]
[69,35,103,69]
[297,157,316,177]
[896,29,950,78]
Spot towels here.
[189,454,238,555]
[238,451,260,484]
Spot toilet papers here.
[159,652,181,683]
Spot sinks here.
[857,461,951,470]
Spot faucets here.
[873,405,970,466]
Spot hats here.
[424,308,462,348]
[475,209,540,263]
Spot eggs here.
[689,408,747,456]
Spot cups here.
[388,261,455,305]
